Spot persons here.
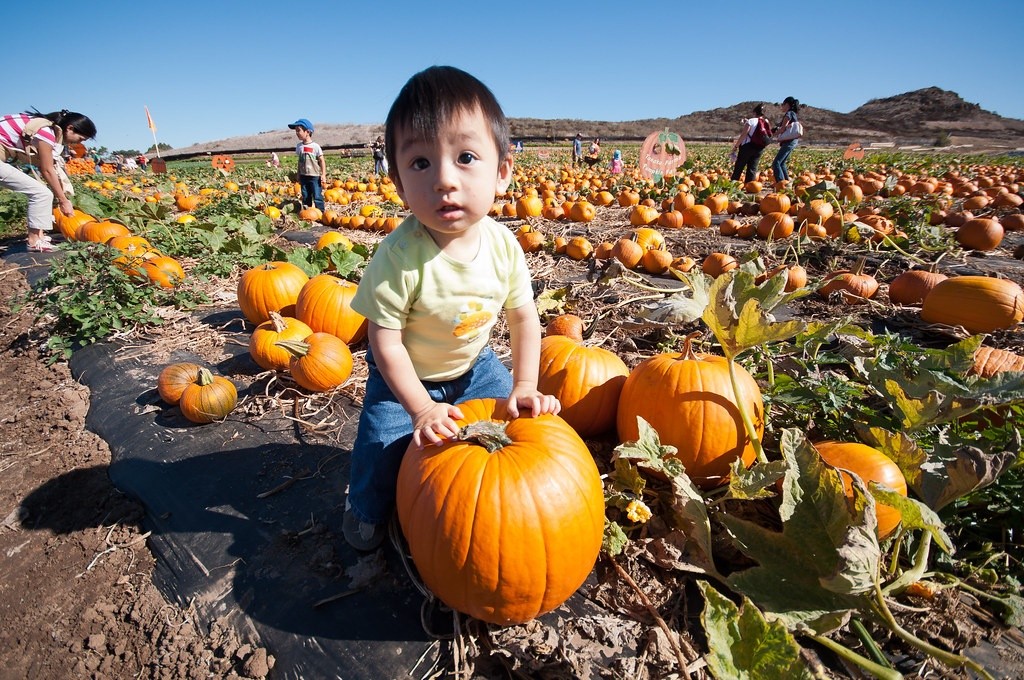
[288,119,326,214]
[371,136,388,177]
[588,138,601,158]
[341,65,560,552]
[341,148,357,157]
[606,150,624,174]
[771,96,799,184]
[265,152,280,168]
[572,133,583,168]
[730,102,773,185]
[513,138,523,153]
[60,145,148,175]
[0,106,97,252]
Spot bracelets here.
[735,146,738,148]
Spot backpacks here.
[748,116,773,146]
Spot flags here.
[145,105,158,132]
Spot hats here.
[288,119,314,133]
[614,150,621,160]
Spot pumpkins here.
[52,157,1024,624]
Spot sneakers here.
[26,239,59,252]
[39,236,52,242]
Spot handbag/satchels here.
[777,112,804,141]
